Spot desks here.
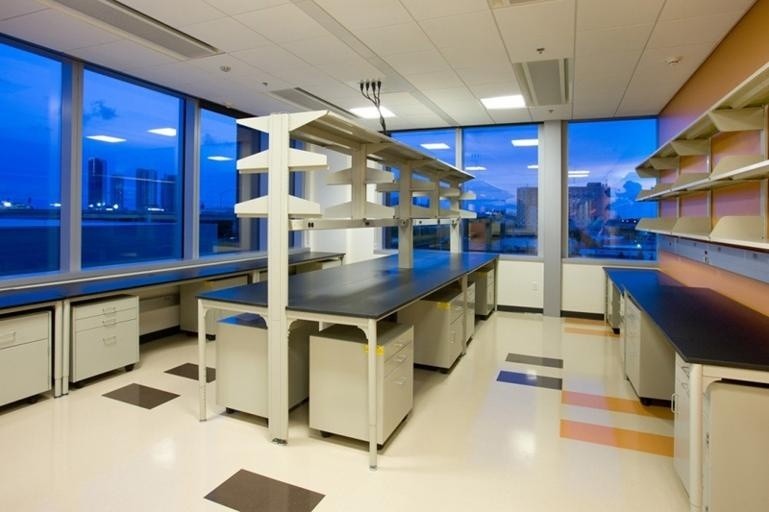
[196,110,499,469]
[622,284,769,512]
[603,266,688,374]
[0,252,346,413]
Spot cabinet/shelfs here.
[634,61,769,250]
[215,268,494,449]
[180,275,248,340]
[670,352,769,512]
[296,259,341,274]
[69,294,138,388]
[625,296,674,406]
[0,310,52,407]
[605,274,620,333]
